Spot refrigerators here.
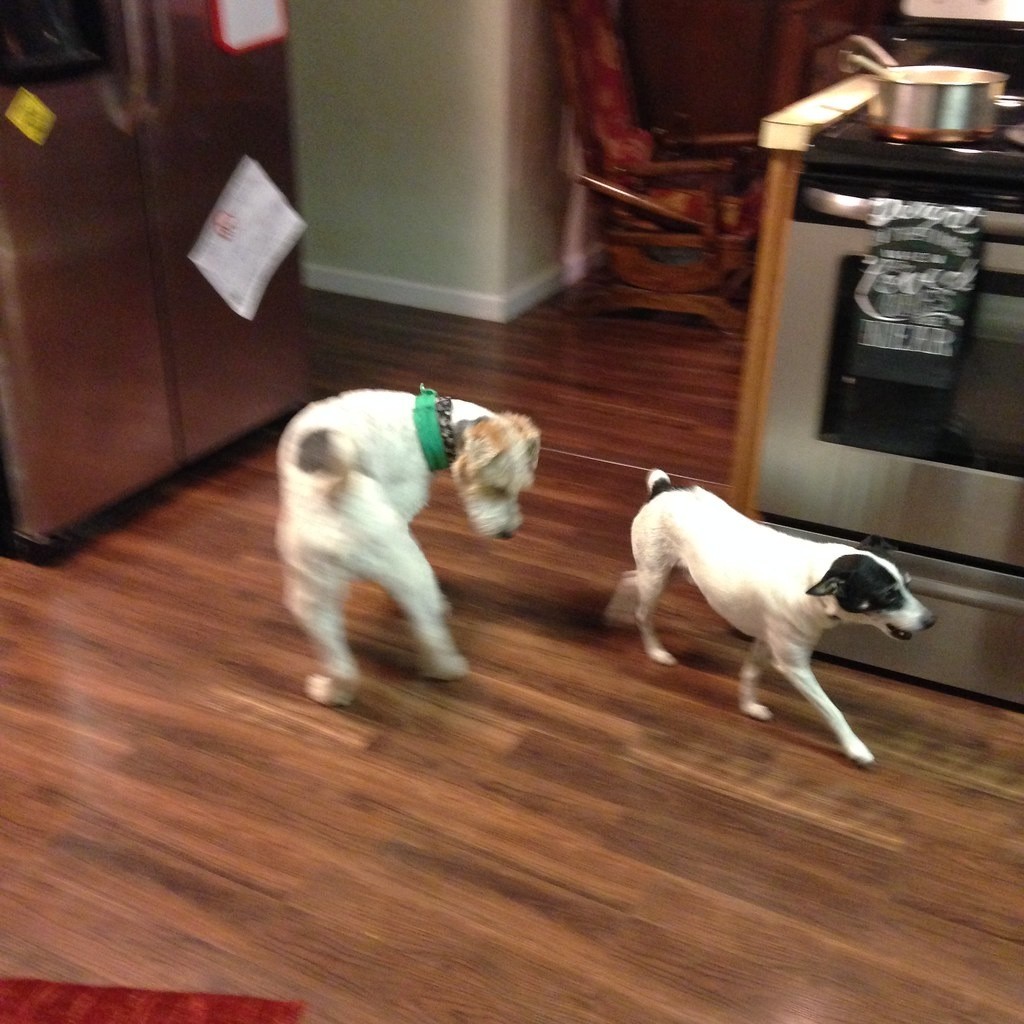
[0,0,327,567]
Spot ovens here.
[750,179,1024,708]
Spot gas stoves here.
[756,19,1024,185]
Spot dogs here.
[274,385,543,708]
[627,465,940,769]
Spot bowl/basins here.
[836,34,1024,145]
